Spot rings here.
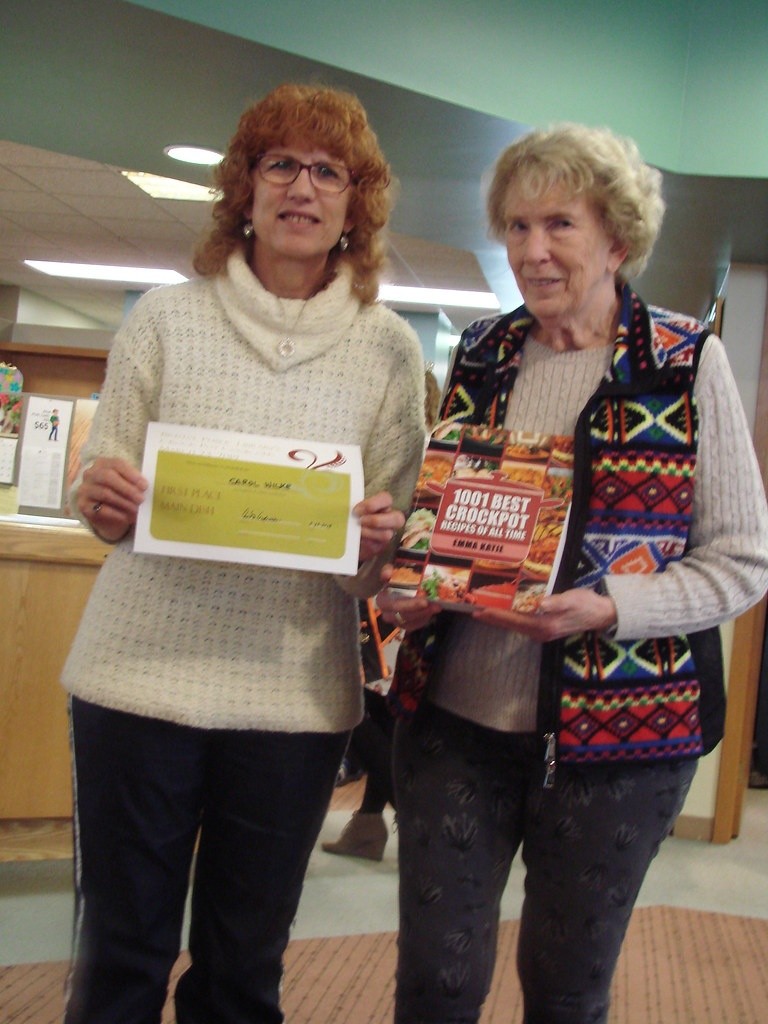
[394,612,407,625]
[91,501,105,518]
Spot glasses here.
[255,154,357,194]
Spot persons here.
[321,587,418,861]
[746,601,768,790]
[63,85,431,1024]
[374,121,768,1024]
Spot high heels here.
[392,812,399,833]
[322,809,388,861]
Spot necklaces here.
[252,256,330,357]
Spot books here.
[388,419,577,615]
